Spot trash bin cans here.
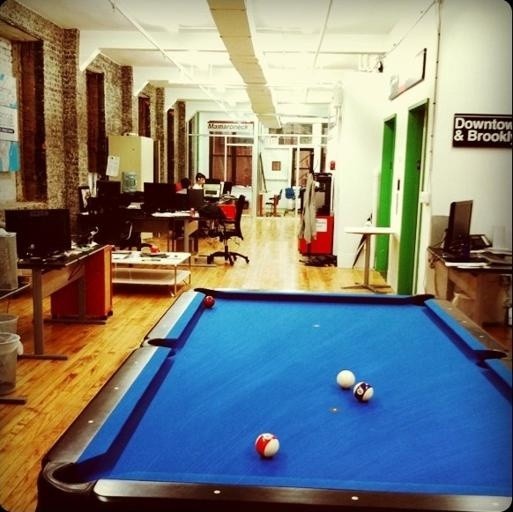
[0,332,21,395]
[0,313,19,334]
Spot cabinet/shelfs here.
[108,135,154,183]
[300,173,332,214]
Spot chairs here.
[207,195,250,266]
[169,192,208,263]
[266,195,279,217]
[269,189,282,201]
[284,188,299,216]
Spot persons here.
[193,173,206,190]
[175,178,191,195]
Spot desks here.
[16,245,113,361]
[76,209,199,263]
[341,227,396,293]
[428,247,512,328]
[37,286,513,512]
[0,282,32,406]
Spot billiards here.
[336,369,356,388]
[204,295,215,307]
[255,433,280,456]
[353,381,374,401]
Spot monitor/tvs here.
[203,183,220,201]
[187,188,204,209]
[143,182,177,213]
[447,200,473,255]
[4,208,71,262]
[204,178,220,185]
[223,181,233,197]
[96,180,121,215]
